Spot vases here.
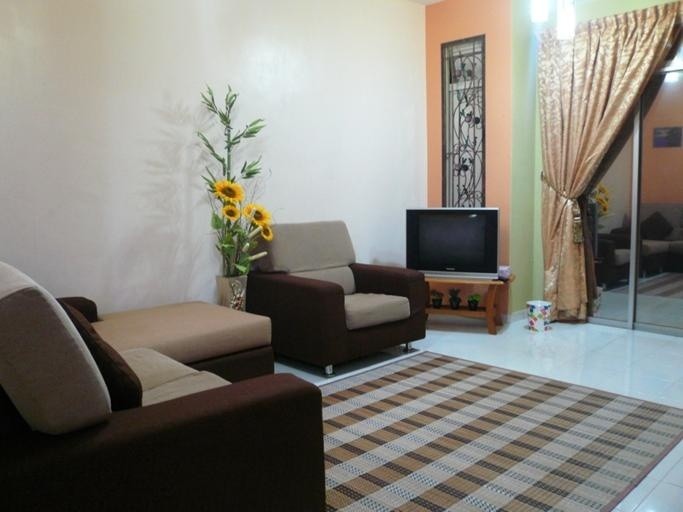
[215,275,248,313]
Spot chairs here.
[246,219,428,378]
[598,211,629,285]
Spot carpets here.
[609,271,683,299]
[317,349,682,512]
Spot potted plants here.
[431,290,444,309]
[448,288,461,310]
[467,294,480,311]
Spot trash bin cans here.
[526,300,552,332]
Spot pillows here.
[57,298,143,411]
[612,227,629,248]
[641,212,673,240]
[56,296,98,323]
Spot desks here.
[426,272,516,334]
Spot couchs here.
[624,203,683,278]
[0,256,325,512]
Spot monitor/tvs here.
[405,209,499,281]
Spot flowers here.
[193,81,274,277]
[590,182,611,229]
